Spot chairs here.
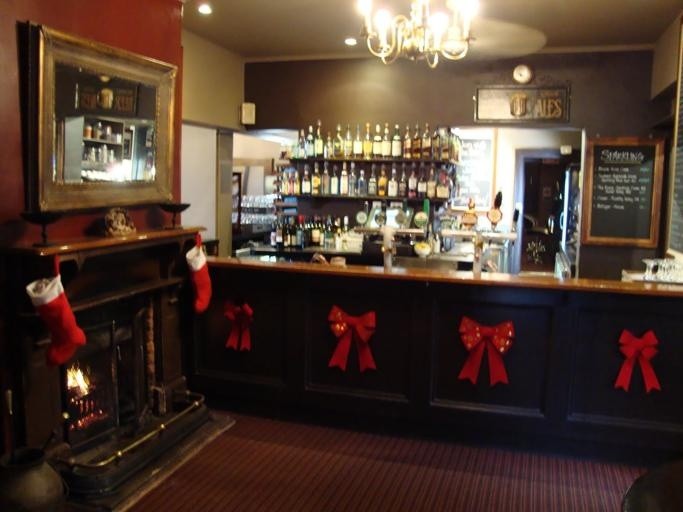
[522,214,550,266]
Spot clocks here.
[512,63,534,84]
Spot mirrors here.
[30,22,179,216]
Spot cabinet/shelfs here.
[62,112,125,184]
[275,157,461,202]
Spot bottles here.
[391,123,403,158]
[348,161,358,196]
[408,161,417,198]
[377,163,388,196]
[344,122,352,160]
[340,161,349,196]
[238,210,277,226]
[333,122,344,158]
[311,161,322,196]
[330,162,339,196]
[301,163,311,195]
[323,130,333,160]
[359,168,368,196]
[369,163,378,196]
[382,122,392,157]
[403,123,412,158]
[294,170,301,195]
[422,122,432,160]
[432,124,441,160]
[283,213,350,251]
[315,119,323,158]
[352,123,363,159]
[306,121,316,158]
[441,126,461,162]
[270,221,276,247]
[276,222,283,248]
[418,162,427,198]
[373,125,382,159]
[240,193,276,209]
[399,162,408,197]
[321,160,330,195]
[427,163,437,198]
[388,161,398,197]
[83,121,113,163]
[282,170,288,196]
[413,121,422,158]
[299,127,306,159]
[436,164,449,198]
[288,167,294,195]
[363,123,372,159]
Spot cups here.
[95,89,112,109]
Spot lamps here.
[343,1,483,70]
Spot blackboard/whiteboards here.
[581,135,665,249]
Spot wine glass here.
[641,257,678,283]
